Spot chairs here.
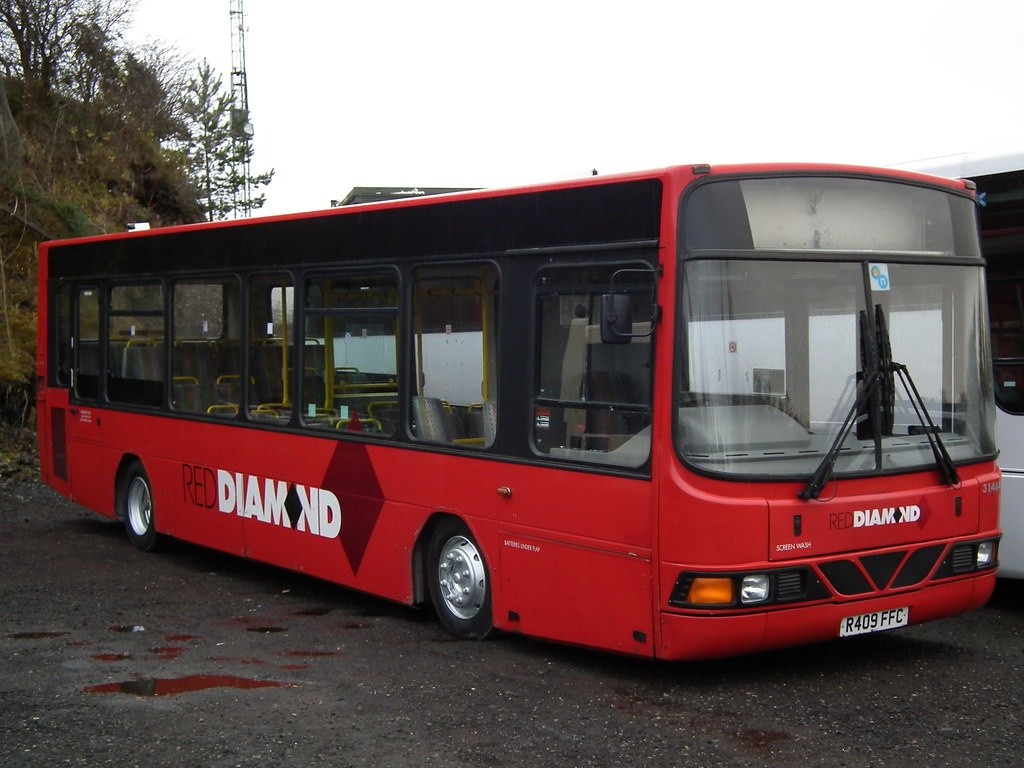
[72,322,537,450]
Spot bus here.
[34,162,1006,679]
[303,159,1023,580]
